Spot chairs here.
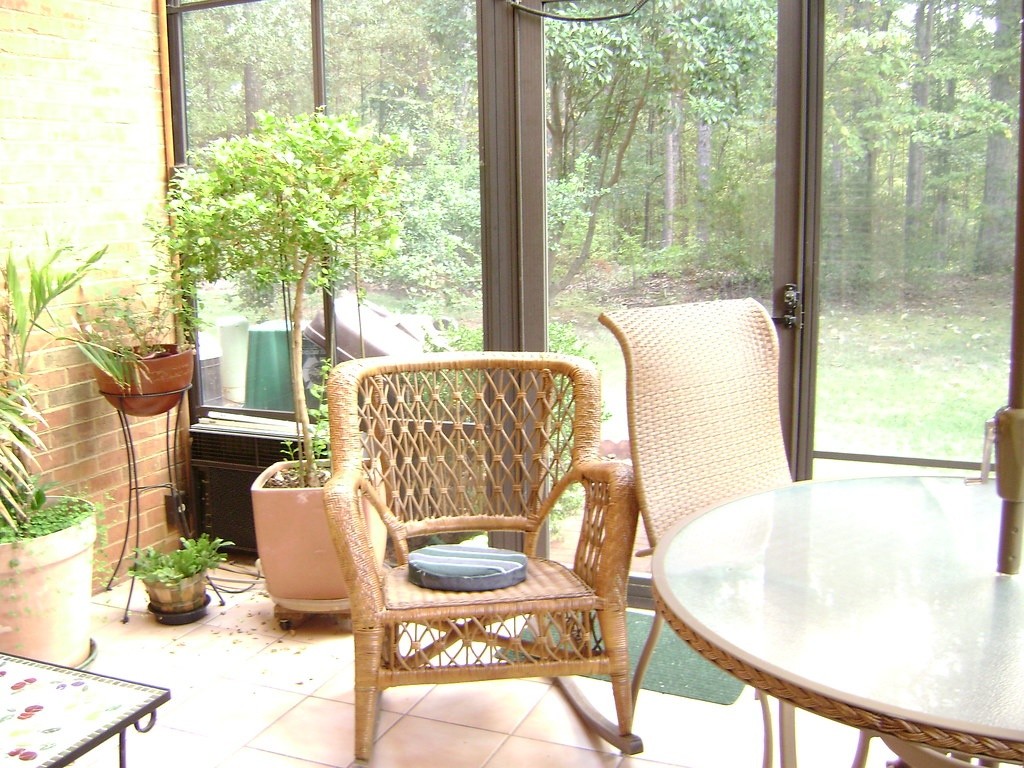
[317,351,644,760]
[598,294,794,768]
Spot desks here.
[649,472,1024,768]
[0,653,171,768]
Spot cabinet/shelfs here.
[97,383,224,624]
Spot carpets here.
[492,587,745,705]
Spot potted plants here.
[0,237,156,670]
[129,534,236,625]
[75,286,197,416]
[158,104,414,615]
